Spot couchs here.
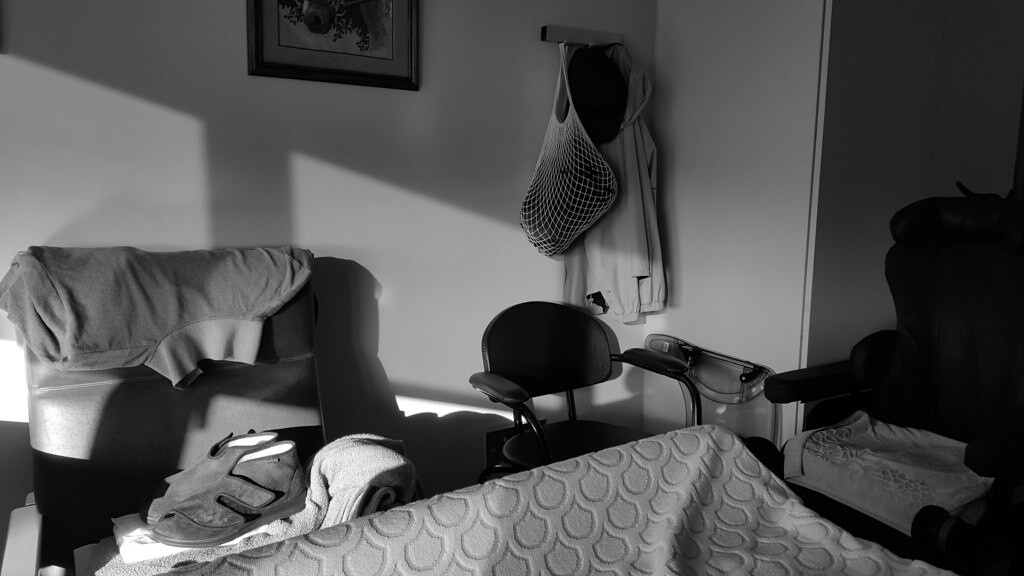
[0,246,416,576]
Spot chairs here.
[469,301,702,484]
[765,197,1024,576]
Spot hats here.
[563,46,628,145]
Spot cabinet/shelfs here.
[643,0,1024,450]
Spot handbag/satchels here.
[519,43,618,257]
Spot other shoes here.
[150,441,307,547]
[139,431,278,525]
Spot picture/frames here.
[246,0,419,91]
